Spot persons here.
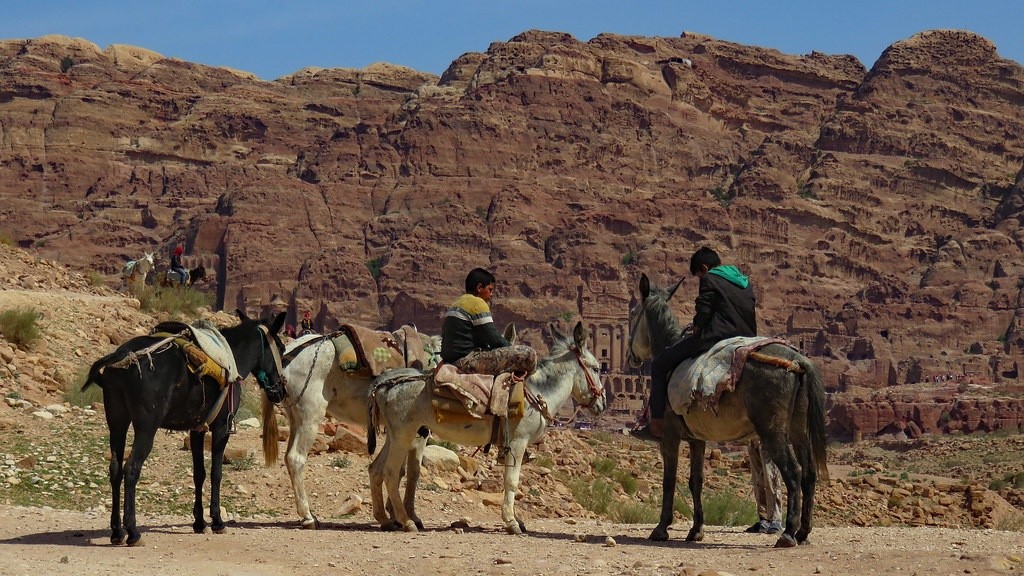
[442,267,538,383]
[171,246,187,287]
[628,245,757,443]
[298,312,317,337]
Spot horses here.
[121,251,156,298]
[263,323,443,532]
[628,272,828,549]
[79,306,291,548]
[150,261,208,297]
[368,320,608,537]
